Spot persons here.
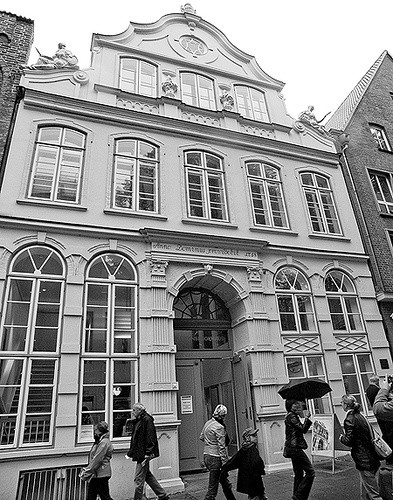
[81,421,114,500]
[200,405,237,500]
[339,395,383,500]
[221,427,269,500]
[284,399,316,500]
[127,403,170,500]
[366,374,393,465]
[82,407,127,438]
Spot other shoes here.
[158,495,171,500]
[292,495,300,500]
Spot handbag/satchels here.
[78,467,94,483]
[372,426,392,457]
[283,440,291,458]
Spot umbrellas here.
[278,376,333,411]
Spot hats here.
[370,375,380,382]
[214,404,227,415]
[242,427,259,437]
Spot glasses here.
[249,433,257,437]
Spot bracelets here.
[306,414,309,418]
